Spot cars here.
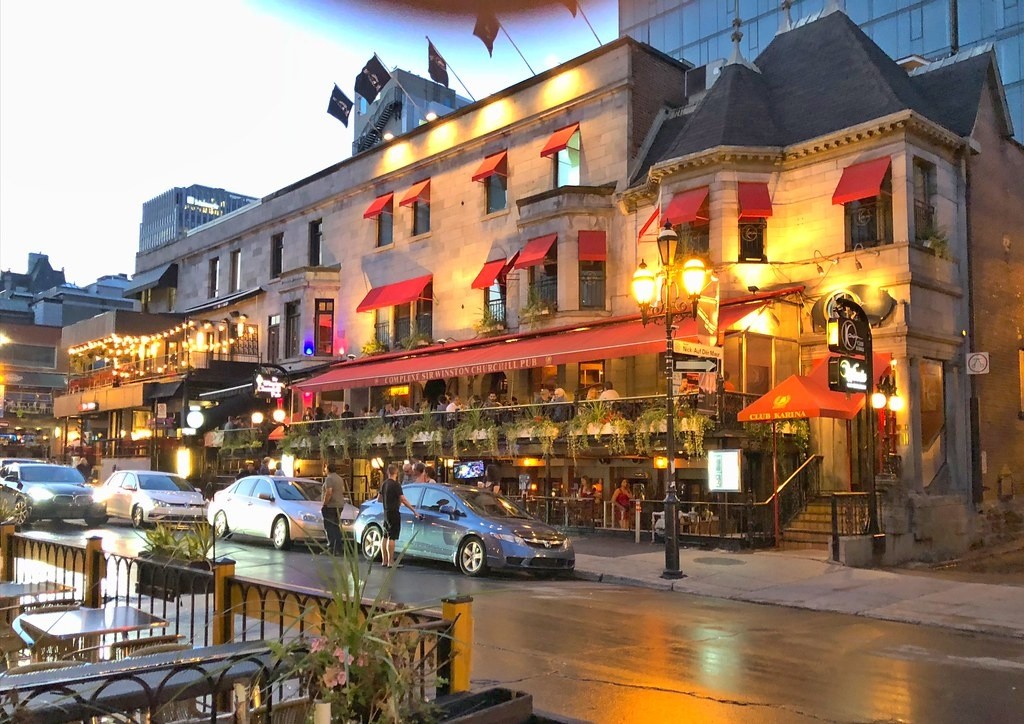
[352,482,576,578]
[98,469,210,530]
[0,462,104,528]
[0,458,50,469]
[207,475,362,550]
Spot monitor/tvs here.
[453,461,485,479]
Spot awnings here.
[363,192,393,220]
[540,122,581,159]
[499,250,521,281]
[356,274,433,313]
[738,182,773,222]
[292,293,771,391]
[832,157,891,207]
[471,151,506,184]
[736,349,890,550]
[150,381,184,399]
[268,412,302,441]
[471,259,506,290]
[121,263,177,300]
[558,0,579,18]
[659,186,710,228]
[399,179,431,208]
[199,377,256,400]
[577,231,607,260]
[514,233,557,270]
[638,207,659,242]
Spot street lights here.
[630,220,719,578]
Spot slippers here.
[381,564,404,568]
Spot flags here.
[427,42,448,89]
[354,55,391,105]
[472,10,500,58]
[327,85,354,128]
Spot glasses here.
[405,468,411,473]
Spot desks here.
[0,639,310,724]
[688,515,720,533]
[20,605,172,667]
[0,581,75,668]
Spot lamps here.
[437,336,460,346]
[814,250,840,275]
[347,353,356,361]
[853,243,880,269]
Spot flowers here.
[187,503,466,724]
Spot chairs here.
[0,598,311,724]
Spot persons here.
[376,464,420,568]
[302,381,619,435]
[223,414,254,445]
[320,464,344,557]
[578,475,637,530]
[401,461,437,486]
[234,456,285,482]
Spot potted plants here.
[399,331,431,347]
[520,285,554,320]
[922,220,949,249]
[136,514,215,594]
[362,338,386,353]
[472,304,505,334]
